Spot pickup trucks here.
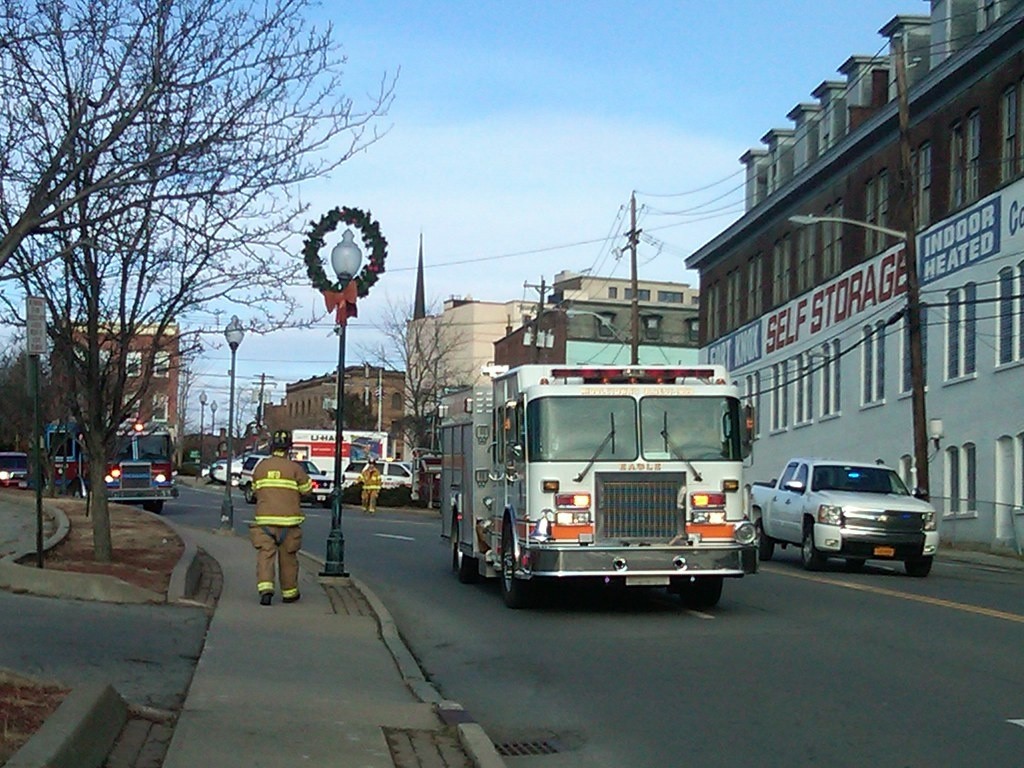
[751,458,940,577]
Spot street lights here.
[210,401,218,458]
[316,228,360,587]
[788,214,934,503]
[196,390,208,481]
[215,315,245,534]
[564,307,642,365]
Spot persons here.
[250,430,315,606]
[360,457,381,514]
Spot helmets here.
[270,429,294,449]
[368,458,376,464]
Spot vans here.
[340,460,414,496]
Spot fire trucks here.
[437,360,761,609]
[40,419,180,514]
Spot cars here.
[211,459,243,487]
[0,450,28,489]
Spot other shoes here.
[282,593,300,602]
[260,594,271,605]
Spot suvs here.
[238,454,334,508]
